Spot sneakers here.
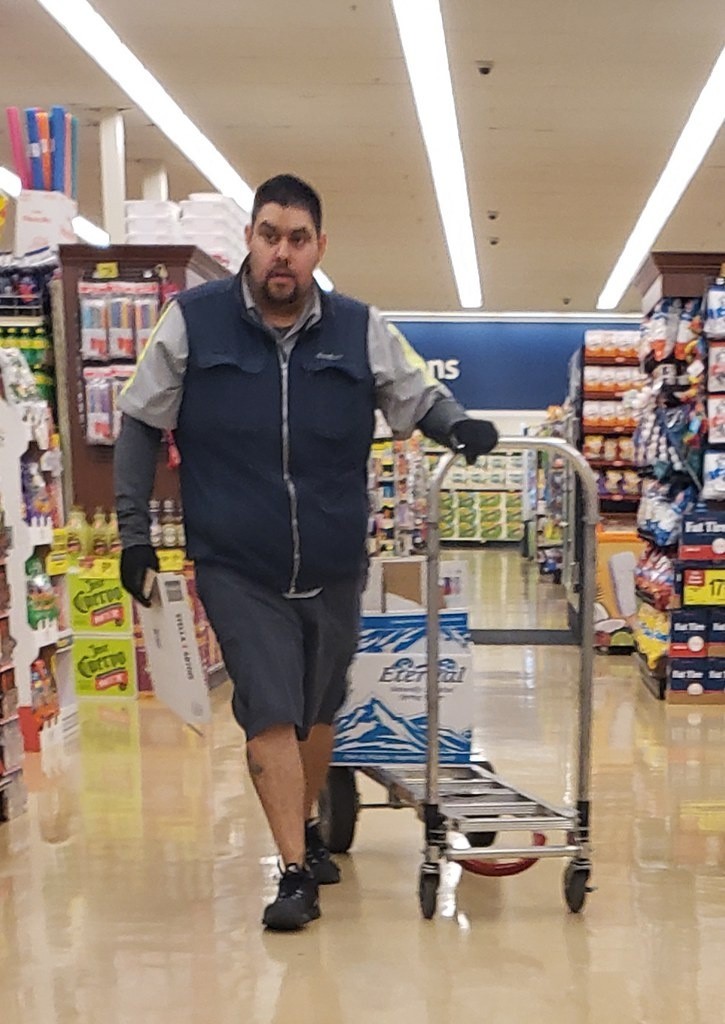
[304,836,340,882]
[264,863,321,928]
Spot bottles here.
[63,498,188,558]
[0,268,54,415]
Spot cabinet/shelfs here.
[51,244,235,690]
[366,251,725,705]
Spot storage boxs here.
[64,548,227,701]
[330,611,473,767]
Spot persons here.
[113,173,499,929]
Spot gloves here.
[447,420,500,464]
[120,545,160,608]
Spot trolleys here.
[314,437,599,927]
